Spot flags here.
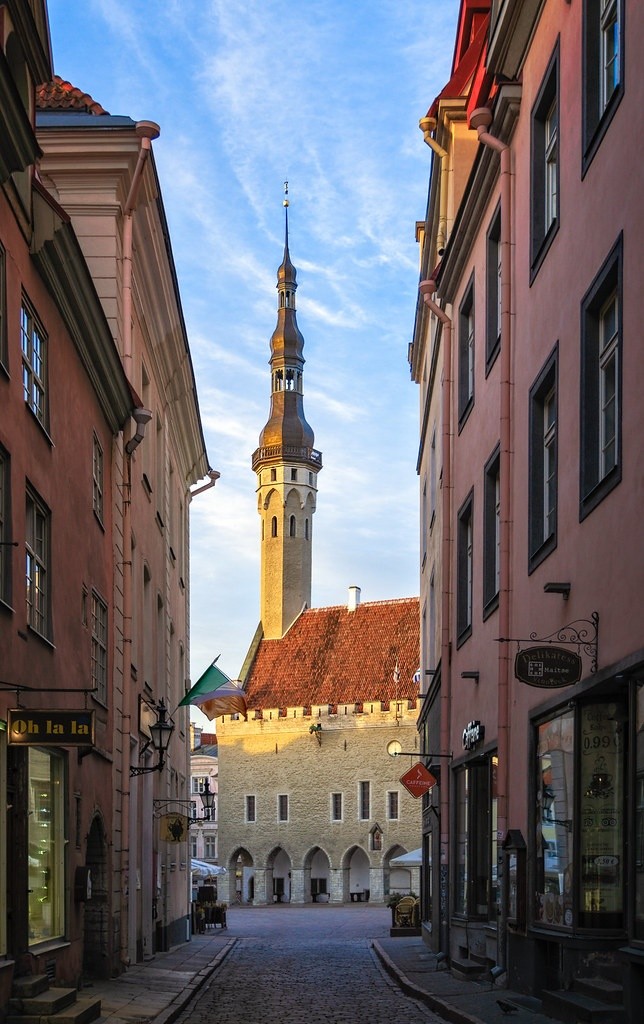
[177,663,248,721]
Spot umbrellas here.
[390,847,423,870]
[191,859,228,877]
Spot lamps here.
[153,777,215,830]
[129,696,175,778]
